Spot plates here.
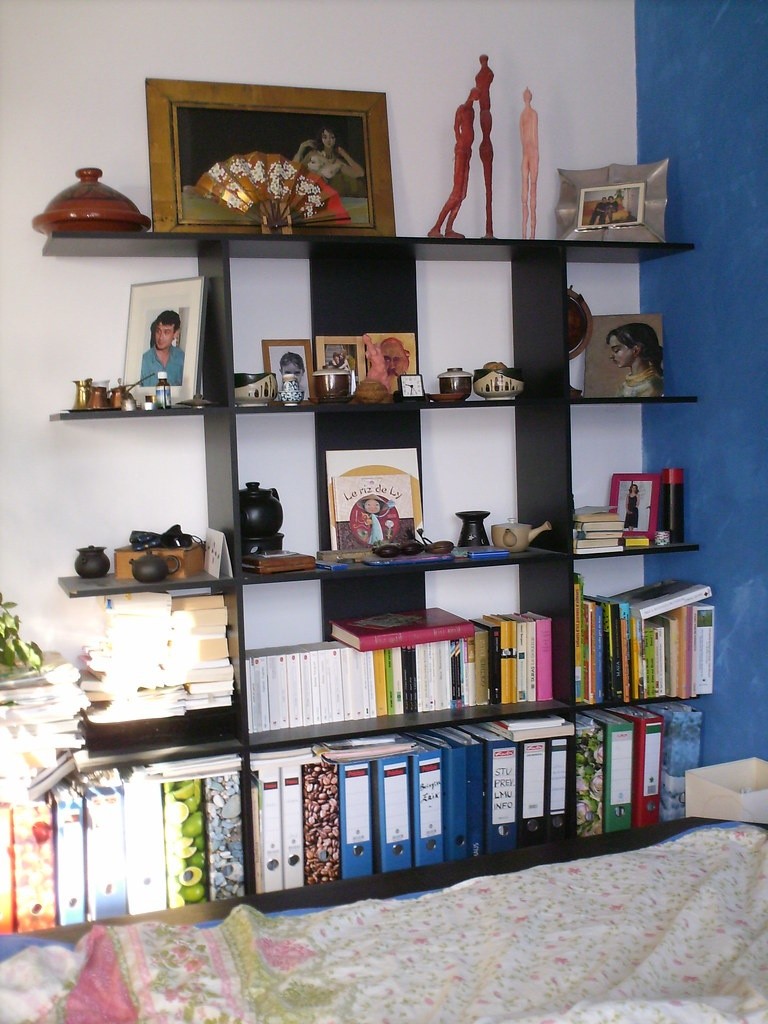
[428,393,463,402]
[308,395,354,403]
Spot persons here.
[427,87,480,238]
[624,484,642,530]
[276,351,307,400]
[140,309,183,386]
[589,194,629,225]
[520,87,540,239]
[328,349,350,371]
[475,55,499,238]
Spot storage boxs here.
[114,541,204,580]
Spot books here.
[573,502,651,556]
[2,587,726,940]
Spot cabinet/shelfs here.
[16,228,715,945]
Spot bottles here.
[156,372,171,408]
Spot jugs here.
[491,518,552,552]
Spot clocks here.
[393,374,425,403]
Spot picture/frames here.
[556,159,670,243]
[315,335,366,402]
[145,78,396,238]
[609,473,662,541]
[261,339,315,407]
[122,276,205,407]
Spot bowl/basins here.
[473,370,523,400]
[438,368,472,400]
[312,365,351,397]
[234,373,278,406]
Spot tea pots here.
[129,550,179,583]
[75,545,110,577]
[238,481,283,536]
